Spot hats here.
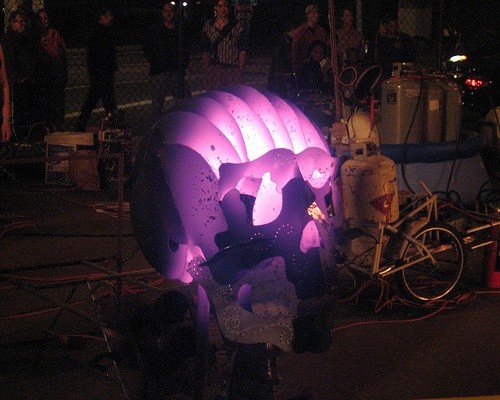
[306,5,319,14]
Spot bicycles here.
[315,178,468,306]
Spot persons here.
[285,6,462,105]
[78,6,119,130]
[203,0,244,89]
[0,42,13,143]
[5,11,73,132]
[148,3,198,123]
